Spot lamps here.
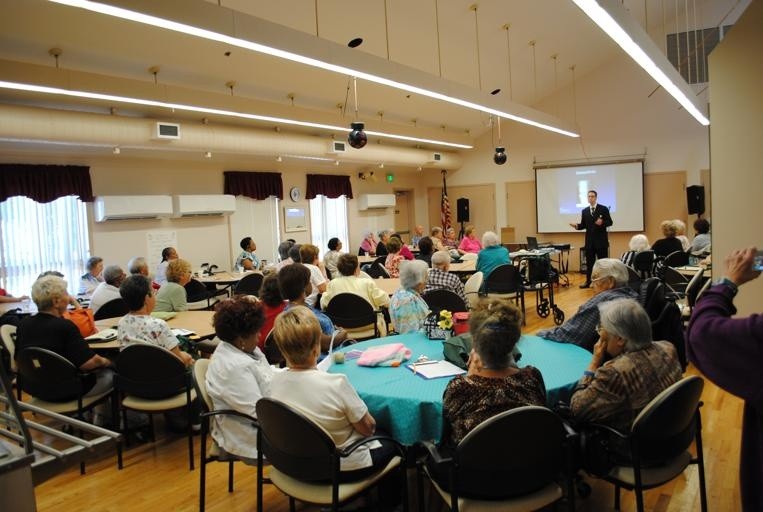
[493,114,507,166]
[0,0,711,159]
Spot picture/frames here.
[283,206,309,233]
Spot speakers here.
[686,185,705,215]
[457,198,469,222]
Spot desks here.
[448,260,477,278]
[409,245,421,252]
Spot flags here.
[440,177,451,232]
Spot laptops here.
[527,237,538,248]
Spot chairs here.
[357,248,391,279]
[478,246,565,326]
[623,249,712,322]
[189,288,707,510]
[0,270,266,474]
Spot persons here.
[568,190,612,288]
[684,246,761,512]
[0,290,28,305]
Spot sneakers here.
[578,280,592,290]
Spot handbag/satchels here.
[60,307,99,338]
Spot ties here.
[591,207,595,217]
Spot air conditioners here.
[170,194,236,219]
[93,194,174,224]
[358,193,395,212]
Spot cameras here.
[751,250,763,272]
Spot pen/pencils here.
[106,333,114,338]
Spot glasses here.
[594,323,604,336]
[147,289,160,296]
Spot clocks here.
[289,186,301,202]
